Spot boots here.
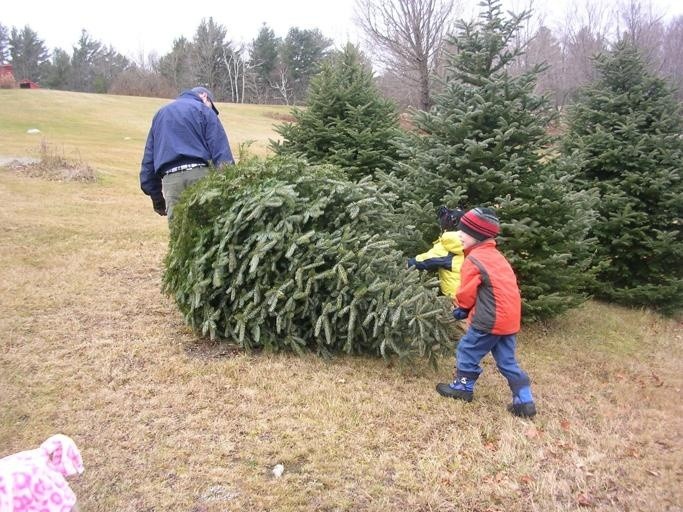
[507,381,536,417]
[436,370,478,402]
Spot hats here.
[441,210,465,232]
[460,207,500,241]
[192,87,219,115]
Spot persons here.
[435,204,537,416]
[404,210,470,332]
[140,86,236,225]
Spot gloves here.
[453,307,469,320]
[153,197,167,216]
[408,256,415,267]
